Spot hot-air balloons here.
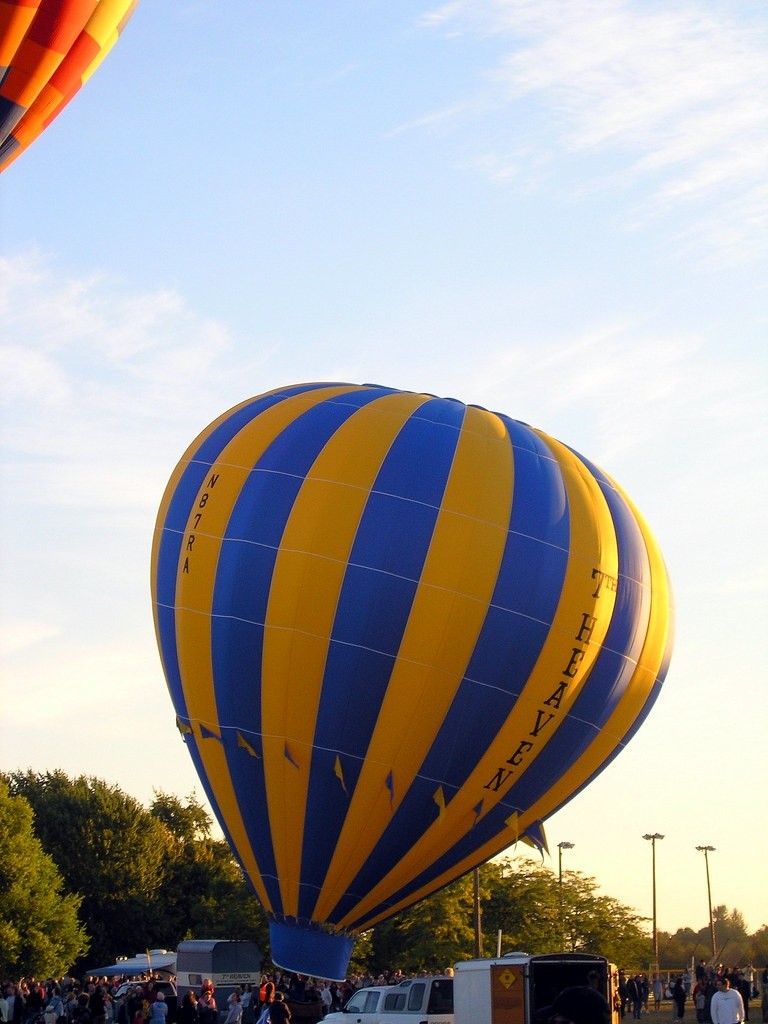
[150,381,675,987]
[0,0,145,175]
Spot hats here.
[157,992,164,1000]
[46,1006,54,1012]
[421,970,427,973]
[319,983,325,987]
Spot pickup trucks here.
[315,976,453,1024]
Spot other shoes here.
[646,1009,650,1014]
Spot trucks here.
[175,939,262,1022]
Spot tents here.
[87,947,177,984]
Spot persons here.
[0,967,453,1024]
[617,961,768,1024]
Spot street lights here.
[556,841,576,954]
[694,845,717,963]
[641,833,666,965]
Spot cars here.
[112,980,179,1024]
